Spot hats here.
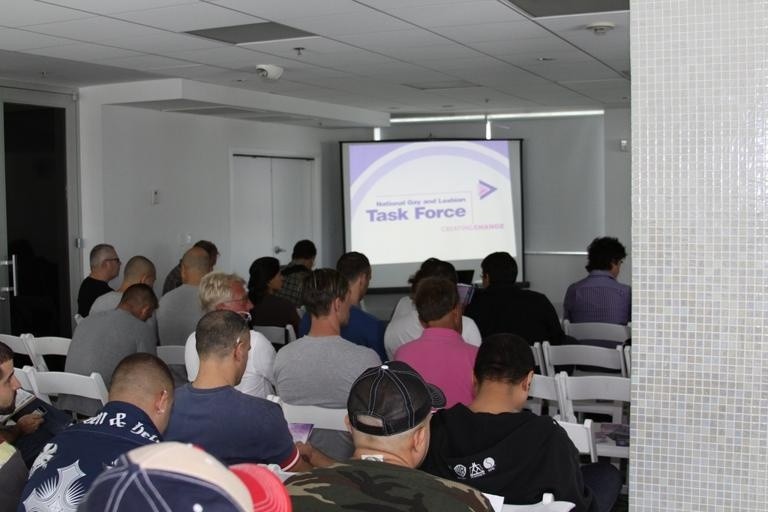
[347,360,447,435]
[76,440,293,512]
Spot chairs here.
[0,309,632,512]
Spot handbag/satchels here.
[4,397,83,460]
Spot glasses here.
[105,258,120,263]
[234,311,252,358]
